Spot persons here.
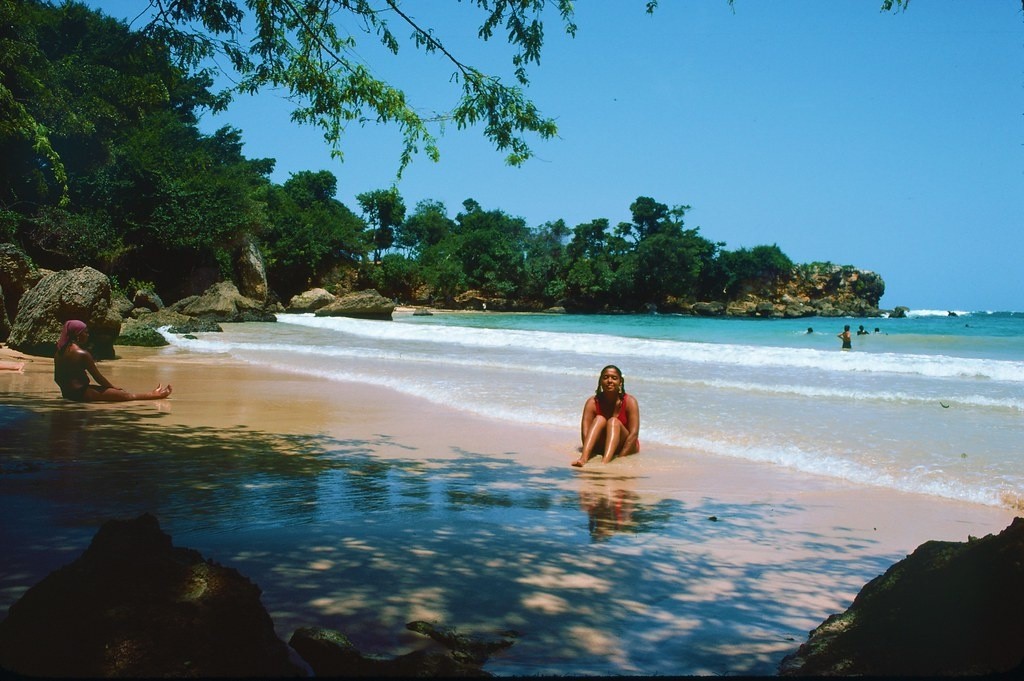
[571,365,640,466]
[875,328,881,333]
[804,327,813,335]
[579,470,633,544]
[856,325,869,335]
[54,320,173,400]
[838,325,851,350]
[0,362,24,370]
